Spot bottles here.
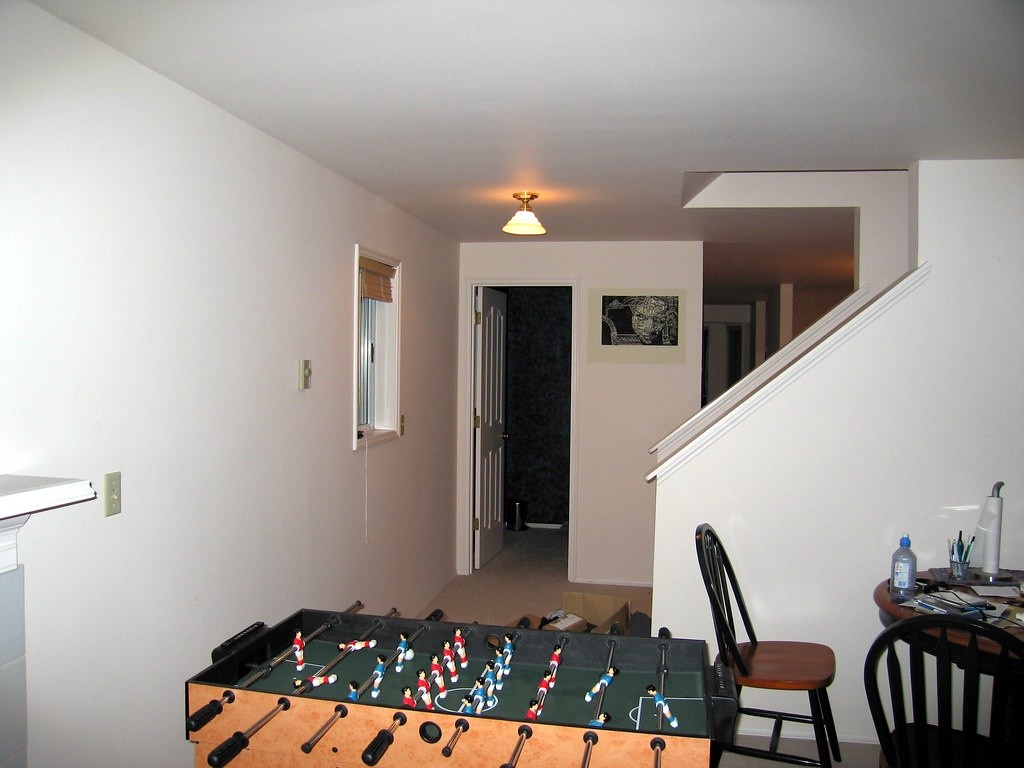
[889,533,917,603]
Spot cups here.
[950,560,970,581]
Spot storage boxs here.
[504,592,632,636]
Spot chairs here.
[863,613,1024,768]
[695,522,842,768]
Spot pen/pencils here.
[914,601,945,614]
[946,530,975,581]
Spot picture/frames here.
[587,286,686,365]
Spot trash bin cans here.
[506,496,529,531]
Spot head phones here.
[914,577,951,594]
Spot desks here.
[873,567,1024,739]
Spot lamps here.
[502,191,548,236]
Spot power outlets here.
[103,472,122,517]
